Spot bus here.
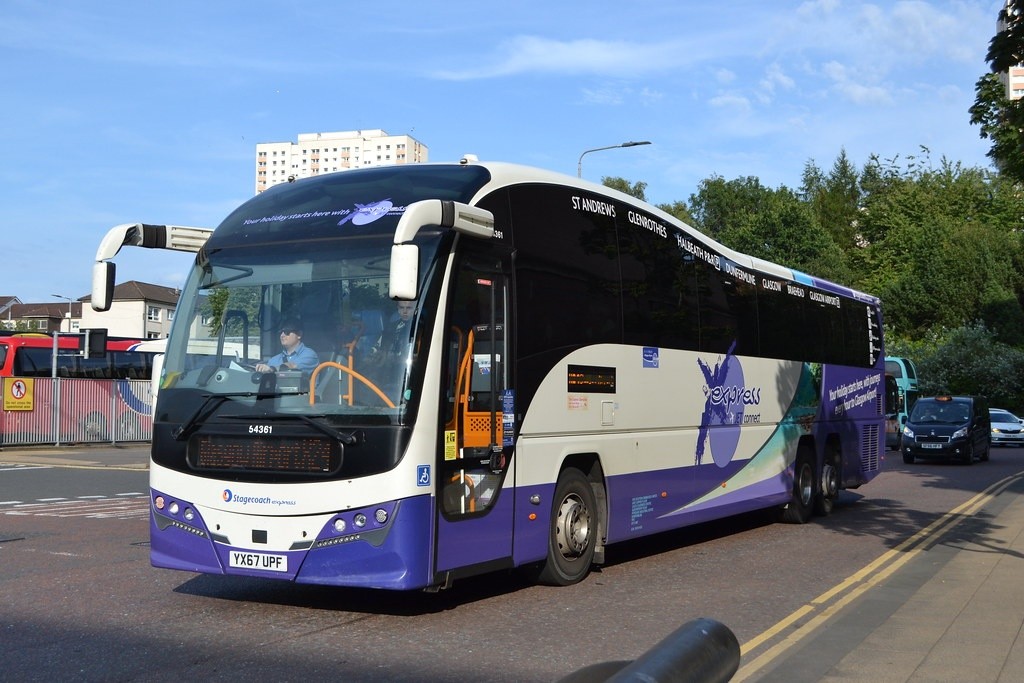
[90,154,888,592]
[884,356,923,450]
[0,333,259,443]
[884,374,904,448]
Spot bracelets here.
[270,366,277,372]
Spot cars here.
[989,408,1024,448]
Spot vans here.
[900,396,991,466]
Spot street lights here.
[578,141,651,179]
[52,295,72,333]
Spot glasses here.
[278,329,295,335]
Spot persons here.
[920,407,936,420]
[369,301,418,384]
[255,319,322,390]
